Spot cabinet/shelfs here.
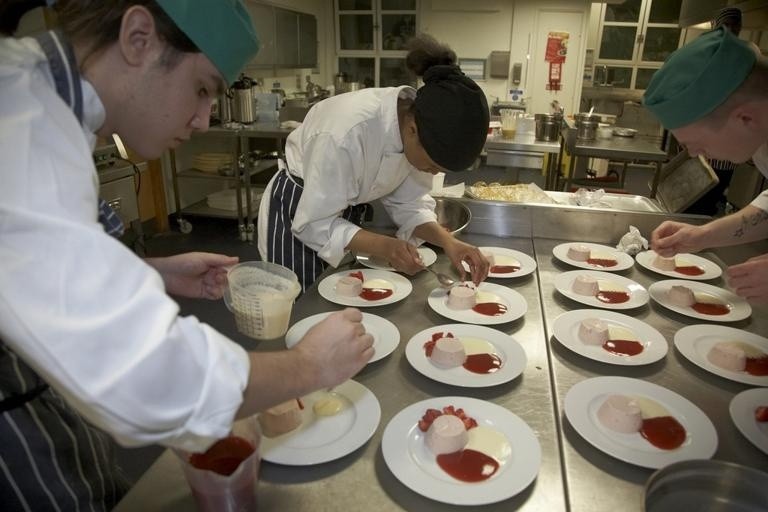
[90,147,154,265]
[232,1,320,76]
[558,119,670,208]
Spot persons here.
[684,7,748,216]
[640,24,768,306]
[1,0,375,512]
[256,31,490,285]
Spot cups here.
[168,420,261,512]
[214,259,304,340]
[499,108,519,138]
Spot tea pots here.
[225,73,260,126]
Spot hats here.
[642,24,757,130]
[716,8,741,25]
[160,0,260,89]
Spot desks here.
[164,106,307,248]
[438,106,563,200]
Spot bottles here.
[335,71,347,90]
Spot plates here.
[193,153,235,176]
[427,280,528,325]
[635,246,768,454]
[612,127,639,137]
[206,188,254,211]
[235,267,414,467]
[551,240,635,273]
[381,395,542,507]
[563,375,718,469]
[553,268,649,310]
[552,308,669,366]
[464,246,538,279]
[405,324,529,388]
[356,243,438,271]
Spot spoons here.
[417,254,455,286]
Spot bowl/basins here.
[432,195,473,238]
[640,456,768,511]
